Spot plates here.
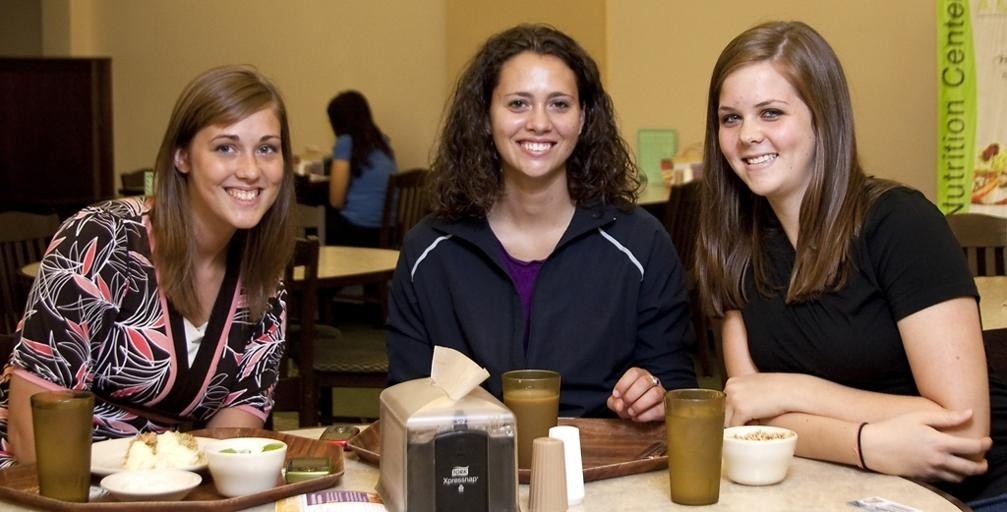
[98,466,204,506]
[90,432,216,470]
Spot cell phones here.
[285,457,331,484]
[319,423,360,451]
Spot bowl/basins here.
[201,438,285,496]
[724,421,798,483]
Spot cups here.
[500,373,560,482]
[29,389,91,504]
[661,390,725,508]
[531,425,584,511]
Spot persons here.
[306,87,402,240]
[690,19,996,483]
[388,22,702,426]
[0,59,296,467]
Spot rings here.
[646,372,661,387]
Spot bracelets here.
[855,418,871,474]
